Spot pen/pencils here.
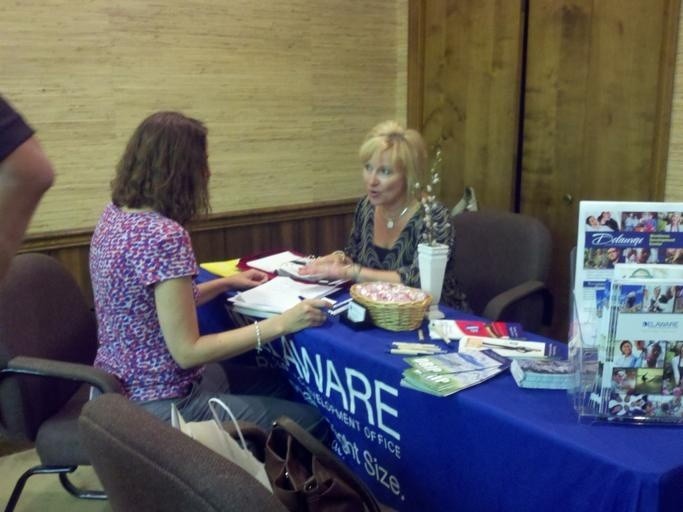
[298,295,336,321]
[433,324,455,347]
[378,339,450,356]
[290,260,307,266]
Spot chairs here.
[78,393,291,512]
[0,252,125,512]
[437,211,554,338]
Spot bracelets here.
[351,263,361,282]
[253,320,264,353]
[331,249,345,264]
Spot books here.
[226,275,338,322]
[238,246,353,287]
[574,200,683,421]
[398,346,515,398]
[509,352,578,391]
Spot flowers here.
[414,149,448,247]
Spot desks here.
[194,267,683,511]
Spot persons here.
[606,246,626,271]
[585,211,618,232]
[616,340,635,368]
[637,343,662,369]
[298,121,476,322]
[664,214,682,233]
[0,90,56,292]
[88,112,334,450]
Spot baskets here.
[349,283,434,331]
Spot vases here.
[416,242,449,310]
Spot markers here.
[418,329,424,342]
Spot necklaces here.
[380,196,414,229]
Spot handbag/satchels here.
[266,414,380,512]
